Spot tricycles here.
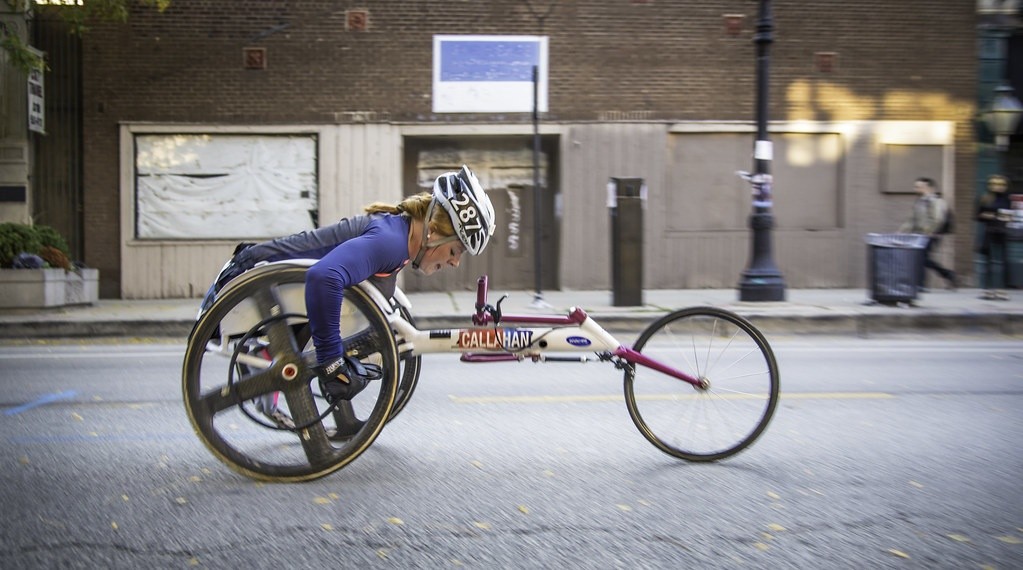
[182,257,780,485]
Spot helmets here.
[434,164,496,256]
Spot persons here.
[898,178,959,290]
[972,174,1013,301]
[194,164,497,405]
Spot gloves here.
[318,355,370,401]
